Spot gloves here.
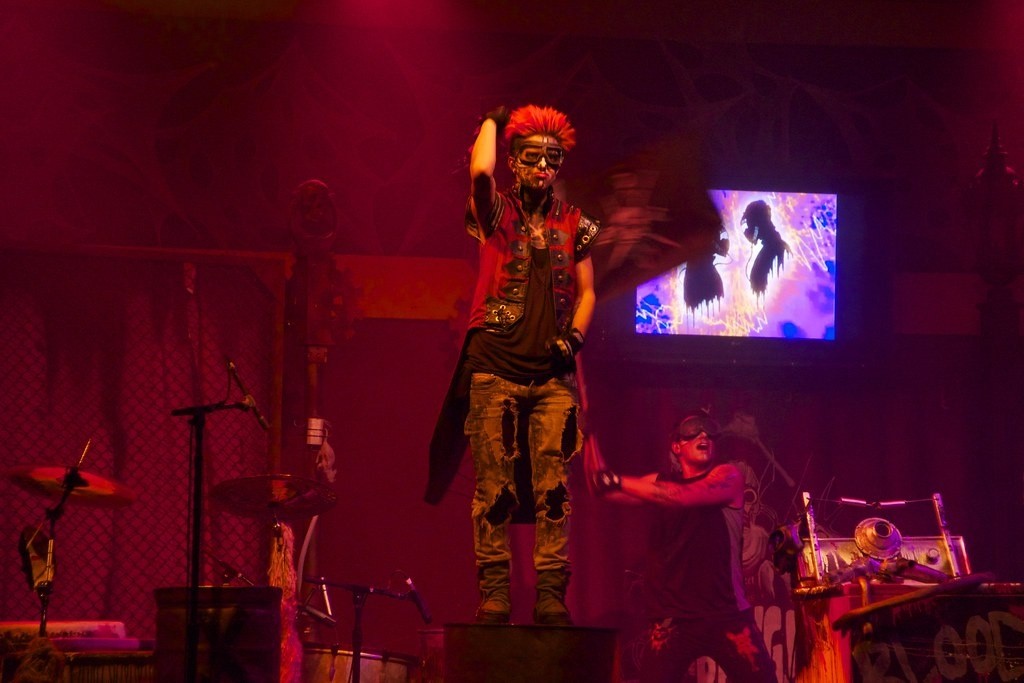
[589,469,621,498]
[545,328,585,359]
[479,106,508,134]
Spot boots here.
[478,561,512,621]
[534,570,574,625]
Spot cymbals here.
[8,465,137,508]
[212,472,334,518]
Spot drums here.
[303,640,422,683]
[153,583,282,683]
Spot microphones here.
[320,576,332,616]
[404,575,432,625]
[227,360,270,432]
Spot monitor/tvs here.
[609,177,862,366]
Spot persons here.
[427,103,602,629]
[585,411,776,683]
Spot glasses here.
[514,144,563,168]
